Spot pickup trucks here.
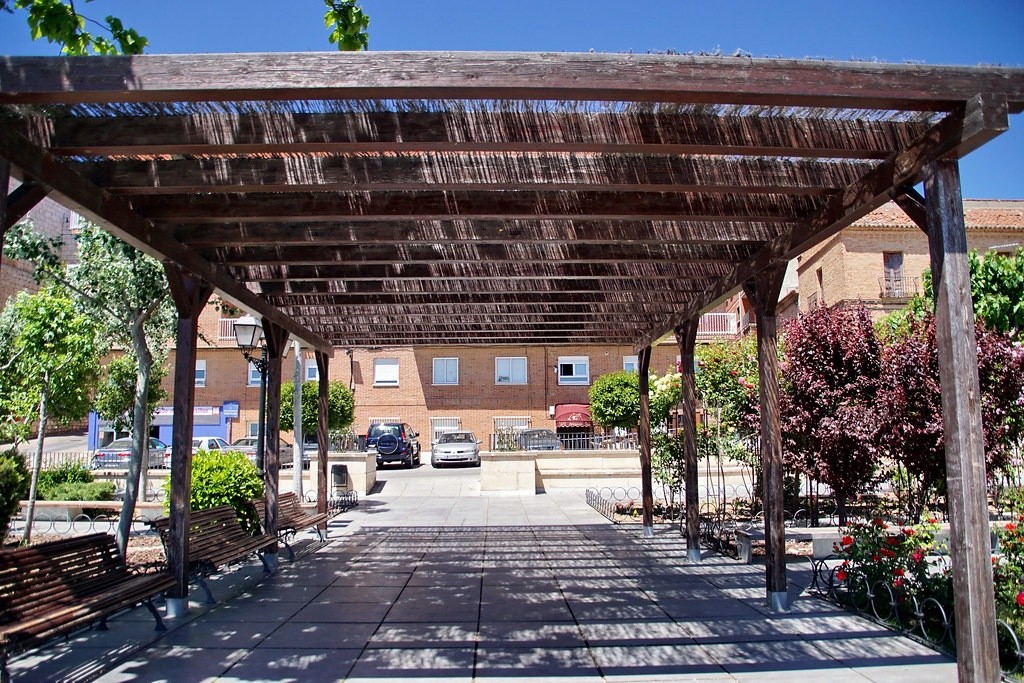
[223,435,294,469]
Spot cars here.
[519,428,565,452]
[430,431,481,468]
[164,437,228,467]
[90,437,166,473]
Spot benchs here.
[732,520,1017,564]
[251,491,332,561]
[18,500,163,523]
[0,531,178,683]
[141,503,280,605]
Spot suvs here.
[365,423,420,467]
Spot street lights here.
[231,313,294,494]
